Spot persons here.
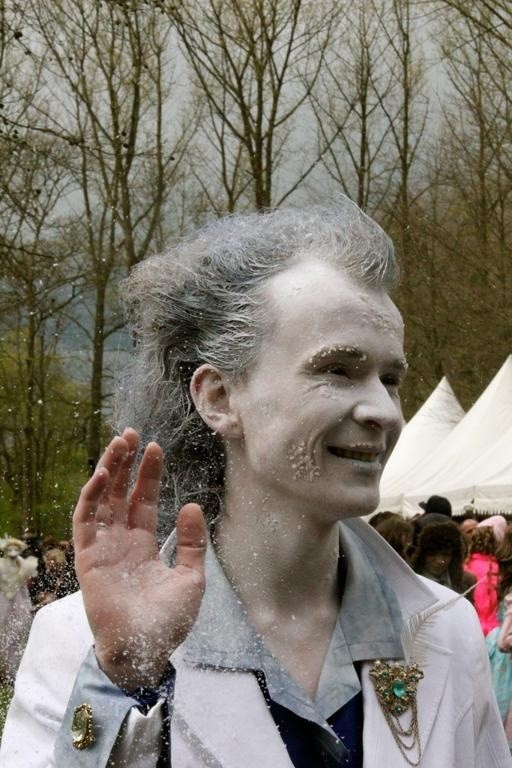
[0,531,79,684]
[0,190,512,768]
[367,493,512,753]
